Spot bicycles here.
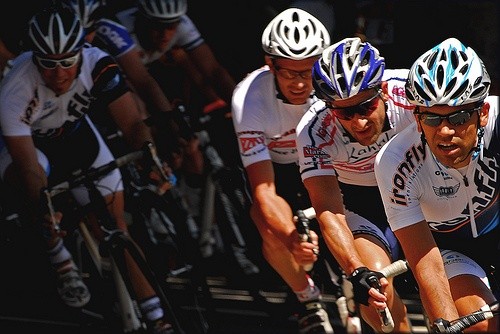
[1,101,499,334]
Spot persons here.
[106,0,259,277]
[0,6,172,334]
[374,37,500,334]
[231,7,362,334]
[295,37,419,334]
[58,0,214,259]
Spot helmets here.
[310,37,386,102]
[260,8,330,61]
[402,37,492,108]
[26,6,85,59]
[141,1,189,24]
[71,0,106,35]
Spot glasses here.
[324,92,381,121]
[414,103,480,126]
[37,52,82,70]
[275,65,315,81]
[153,17,183,30]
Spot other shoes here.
[49,265,92,309]
[287,299,335,334]
